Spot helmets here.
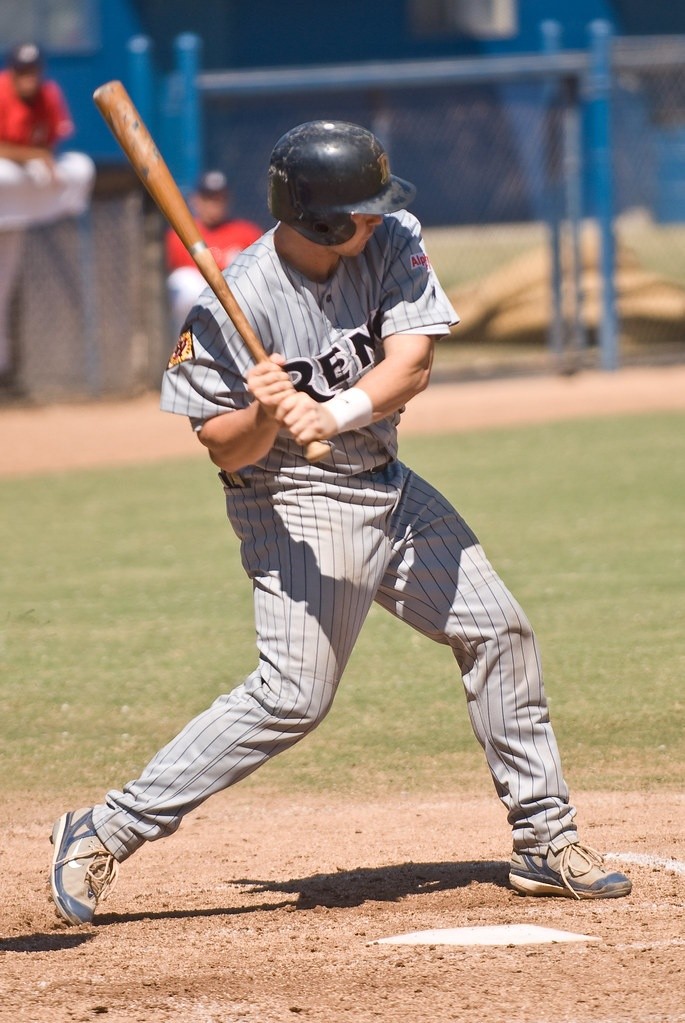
[266,119,416,247]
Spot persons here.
[0,42,121,405]
[46,117,633,929]
[165,169,263,347]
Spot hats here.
[11,42,41,72]
[194,170,228,198]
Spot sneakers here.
[48,806,119,926]
[508,842,632,900]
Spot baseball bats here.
[89,76,334,463]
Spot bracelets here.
[321,385,374,434]
